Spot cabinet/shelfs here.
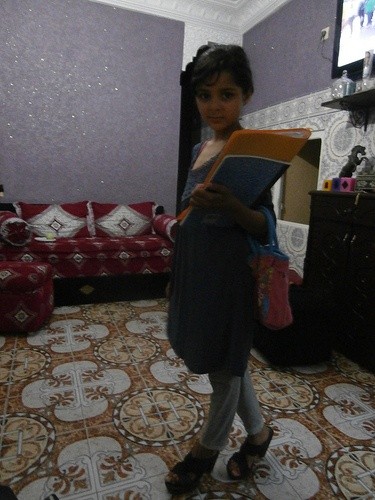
[303,190,375,374]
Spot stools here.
[0,262,54,332]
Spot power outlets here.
[321,27,329,40]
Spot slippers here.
[226,427,274,480]
[166,451,220,493]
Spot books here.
[180,155,291,226]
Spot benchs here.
[0,201,180,297]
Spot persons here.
[358,0,375,27]
[167,44,278,493]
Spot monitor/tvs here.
[331,0,375,78]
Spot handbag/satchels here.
[245,207,293,329]
